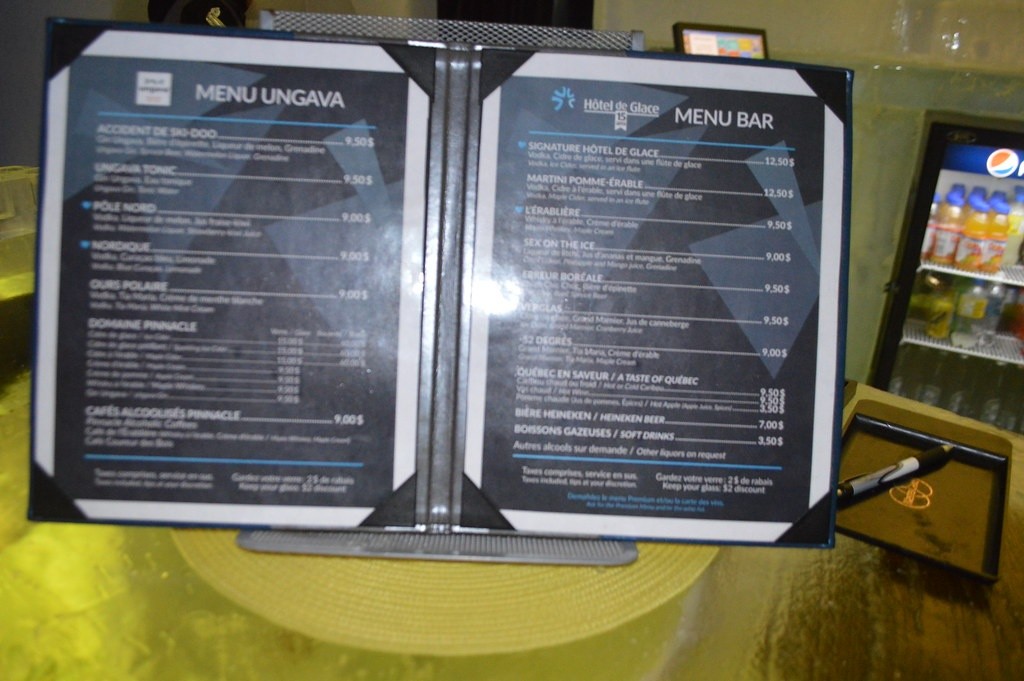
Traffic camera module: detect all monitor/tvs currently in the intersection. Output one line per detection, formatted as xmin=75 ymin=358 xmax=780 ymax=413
xmin=673 ymin=22 xmax=768 ymax=60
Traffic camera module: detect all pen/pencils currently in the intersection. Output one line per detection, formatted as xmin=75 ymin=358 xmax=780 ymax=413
xmin=837 ymin=443 xmax=955 ymax=501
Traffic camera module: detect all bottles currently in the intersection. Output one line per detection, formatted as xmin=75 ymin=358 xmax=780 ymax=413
xmin=879 ymin=183 xmax=1024 ymax=438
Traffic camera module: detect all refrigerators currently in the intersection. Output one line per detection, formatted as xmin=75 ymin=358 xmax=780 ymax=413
xmin=862 ymin=108 xmax=1023 ymax=443
